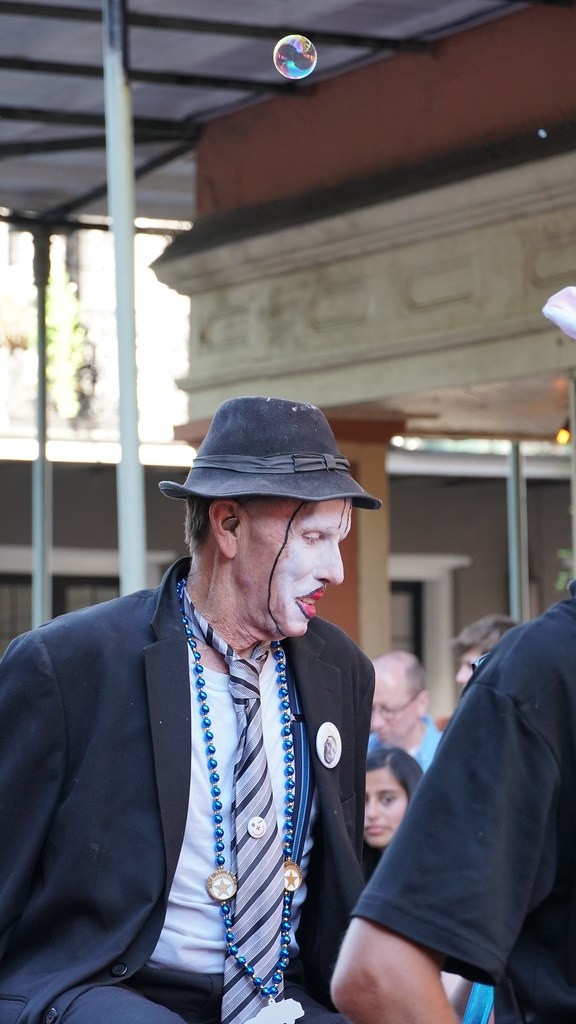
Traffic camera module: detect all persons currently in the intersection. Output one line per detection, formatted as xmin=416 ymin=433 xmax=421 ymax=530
xmin=1 ymin=397 xmax=376 ymax=1023
xmin=325 ymin=573 xmax=571 ymax=1024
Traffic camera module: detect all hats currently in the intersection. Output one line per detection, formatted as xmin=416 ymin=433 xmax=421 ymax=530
xmin=159 ymin=398 xmax=382 ymax=511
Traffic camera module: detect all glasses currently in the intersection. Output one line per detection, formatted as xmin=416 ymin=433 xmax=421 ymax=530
xmin=371 ymin=690 xmax=420 ymax=719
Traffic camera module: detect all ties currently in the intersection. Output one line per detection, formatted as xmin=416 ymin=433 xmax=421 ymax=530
xmin=176 ymin=581 xmax=283 ymax=1023
